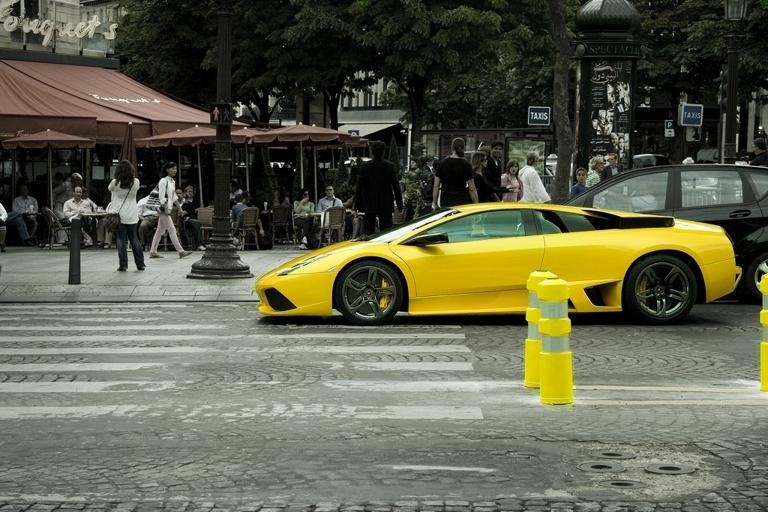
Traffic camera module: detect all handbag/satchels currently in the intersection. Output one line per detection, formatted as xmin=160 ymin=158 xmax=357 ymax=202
xmin=101 ymin=213 xmax=121 ymax=232
xmin=145 ymin=179 xmax=168 ymax=212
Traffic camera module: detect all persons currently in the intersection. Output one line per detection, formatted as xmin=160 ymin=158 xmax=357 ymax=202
xmin=755 ymin=125 xmax=768 ymax=143
xmin=409 ymin=136 xmax=623 ymax=217
xmin=150 ymin=161 xmax=192 ymax=258
xmin=750 ymin=138 xmax=768 ymax=168
xmin=603 ymin=179 xmax=659 ymax=210
xmin=481 ymin=213 xmax=517 ymax=236
xmin=682 ymin=153 xmax=695 ymax=164
xmin=592 ymin=83 xmax=629 ymax=157
xmin=352 ymin=142 xmax=404 ymax=233
xmin=685 ymin=127 xmax=701 ymax=141
xmin=105 ymin=161 xmax=147 ymax=273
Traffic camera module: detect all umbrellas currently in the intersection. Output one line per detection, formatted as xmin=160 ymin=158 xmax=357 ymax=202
xmin=118 ymin=121 xmax=140 ymax=179
xmin=0 ymin=126 xmax=96 ymax=209
xmin=135 ymin=128 xmax=184 ymax=186
xmin=231 ymin=120 xmax=370 ymax=210
xmin=150 ymin=124 xmax=246 ymax=240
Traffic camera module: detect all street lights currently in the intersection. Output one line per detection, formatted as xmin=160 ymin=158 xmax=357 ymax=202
xmin=716 ymin=0 xmax=752 ymax=166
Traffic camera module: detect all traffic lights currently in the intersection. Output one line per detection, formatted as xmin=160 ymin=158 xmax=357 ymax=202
xmin=210 ymin=102 xmax=234 ymax=124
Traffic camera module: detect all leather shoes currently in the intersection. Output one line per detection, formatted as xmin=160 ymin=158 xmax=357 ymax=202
xmin=103 ymin=242 xmax=112 ymax=248
xmin=198 ymin=245 xmax=206 ymax=251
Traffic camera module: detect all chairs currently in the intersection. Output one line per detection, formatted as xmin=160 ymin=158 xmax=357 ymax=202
xmin=0 ymin=188 xmax=407 ymax=251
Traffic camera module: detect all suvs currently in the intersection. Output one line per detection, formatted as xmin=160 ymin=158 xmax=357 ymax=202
xmin=559 ymin=162 xmax=768 ymax=304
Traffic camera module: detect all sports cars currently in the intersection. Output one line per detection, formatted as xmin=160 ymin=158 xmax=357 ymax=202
xmin=251 ymin=200 xmax=748 ymax=326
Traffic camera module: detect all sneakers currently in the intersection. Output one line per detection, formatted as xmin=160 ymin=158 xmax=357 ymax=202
xmin=138 ymin=265 xmax=145 ymax=270
xmin=23 ymin=236 xmax=39 ymax=246
xmin=299 ymin=236 xmax=308 ymax=250
xmin=117 ymin=267 xmax=126 ymax=272
xmin=179 ymin=251 xmax=192 ymax=259
xmin=149 ymin=253 xmax=164 ymax=258
